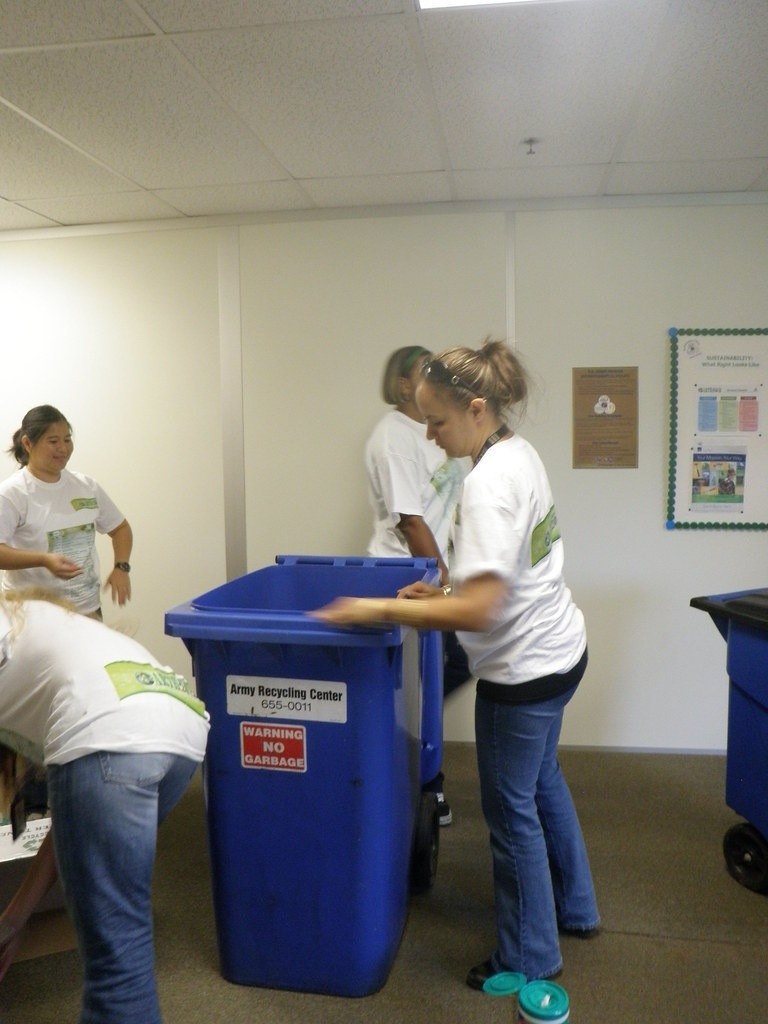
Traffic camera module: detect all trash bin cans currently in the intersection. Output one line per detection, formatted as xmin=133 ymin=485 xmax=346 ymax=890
xmin=689 ymin=585 xmax=768 ymax=896
xmin=162 ymin=552 xmax=444 ymax=1001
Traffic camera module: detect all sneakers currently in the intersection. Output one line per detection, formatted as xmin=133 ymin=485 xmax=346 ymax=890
xmin=435 ymin=785 xmax=452 ymax=825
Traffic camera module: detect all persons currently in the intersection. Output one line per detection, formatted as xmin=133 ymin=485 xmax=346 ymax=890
xmin=308 ymin=341 xmax=601 ymax=992
xmin=0 ymin=589 xmax=210 ymax=1024
xmin=719 ymin=470 xmax=735 ymax=495
xmin=364 ymin=346 xmax=471 ymax=826
xmin=0 ymin=405 xmax=133 ymax=618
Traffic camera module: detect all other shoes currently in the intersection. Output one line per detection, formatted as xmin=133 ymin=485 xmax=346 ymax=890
xmin=467 ymin=950 xmax=565 ymax=992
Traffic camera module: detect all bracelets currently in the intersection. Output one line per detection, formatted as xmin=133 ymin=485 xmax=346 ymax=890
xmin=441 ymin=584 xmax=451 ymax=595
xmin=114 ymin=562 xmax=130 ymax=571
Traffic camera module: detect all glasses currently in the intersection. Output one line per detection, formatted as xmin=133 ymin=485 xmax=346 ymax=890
xmin=418 ymin=357 xmax=483 ymax=398
